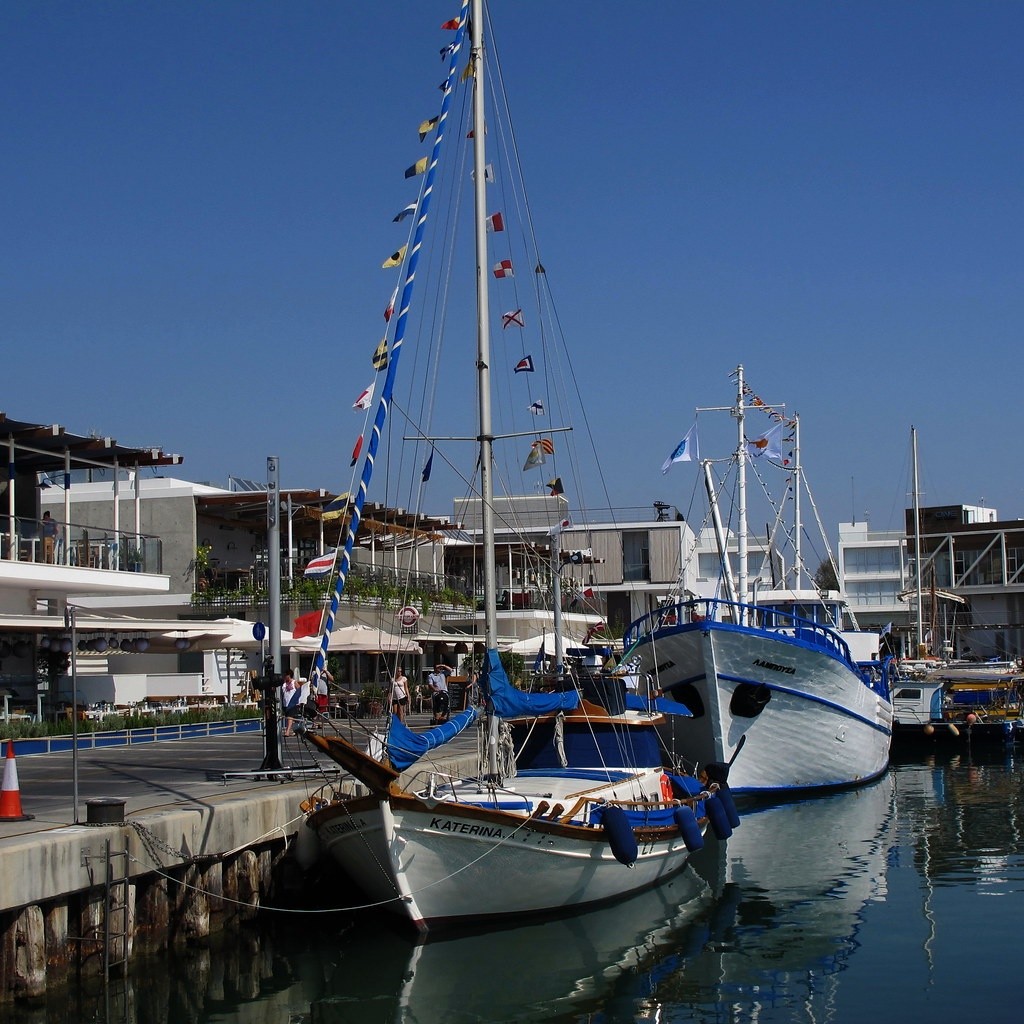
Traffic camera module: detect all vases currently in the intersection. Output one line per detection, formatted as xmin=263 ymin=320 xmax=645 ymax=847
xmin=198 ymin=577 xmax=209 ymax=592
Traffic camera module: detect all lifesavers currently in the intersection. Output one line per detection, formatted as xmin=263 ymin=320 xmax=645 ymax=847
xmin=660 ymin=774 xmax=674 ymax=808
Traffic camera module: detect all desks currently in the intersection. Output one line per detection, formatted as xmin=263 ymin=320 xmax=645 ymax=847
xmin=86 ymin=711 xmax=120 ymax=722
xmin=141 ymin=708 xmax=156 ymax=716
xmin=70 ymin=543 xmax=110 ymax=569
xmin=188 ymin=704 xmax=224 ymax=710
xmin=0 ymin=714 xmax=32 ymax=722
xmin=0 ymin=533 xmax=18 ymax=562
xmin=217 ymin=568 xmax=251 ymax=591
xmin=19 ymin=538 xmax=40 ymax=563
xmin=158 ymin=706 xmax=190 ymax=714
xmin=231 ymin=703 xmax=259 ymax=711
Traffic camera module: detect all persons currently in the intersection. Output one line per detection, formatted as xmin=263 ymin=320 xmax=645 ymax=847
xmin=312 ymin=661 xmax=333 ymax=727
xmin=37 ymin=511 xmax=59 ymax=561
xmin=428 ymin=662 xmax=454 ymax=721
xmin=388 ymin=667 xmax=409 ymax=723
xmin=465 ymin=667 xmax=478 ymax=709
xmin=283 ymin=668 xmax=300 ymax=736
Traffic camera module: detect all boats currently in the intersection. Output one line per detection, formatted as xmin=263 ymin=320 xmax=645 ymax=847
xmin=710 ymin=768 xmax=900 ymax=948
xmin=620 ymin=364 xmax=896 ymax=806
xmin=890 ymin=423 xmax=1023 ymax=735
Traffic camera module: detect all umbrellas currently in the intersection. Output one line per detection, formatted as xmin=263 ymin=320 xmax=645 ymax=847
xmin=139 ymin=615 xmax=321 ymax=707
xmin=263 ymin=620 xmax=424 ymax=693
xmin=505 ymin=631 xmax=590 ymax=660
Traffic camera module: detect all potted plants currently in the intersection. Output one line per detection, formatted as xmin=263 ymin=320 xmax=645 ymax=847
xmin=118 ymin=540 xmax=144 ymax=572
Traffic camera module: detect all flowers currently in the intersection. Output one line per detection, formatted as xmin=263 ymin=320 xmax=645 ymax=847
xmin=196 ymin=543 xmax=213 ymax=566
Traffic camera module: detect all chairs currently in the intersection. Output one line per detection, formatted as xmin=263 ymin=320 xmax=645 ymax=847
xmin=329 ymin=692 xmax=342 ymax=719
xmin=93 ymin=546 xmax=110 ymax=569
xmin=345 ymin=692 xmax=360 ymax=719
xmin=66 ymin=705 xmax=257 ymax=720
xmin=45 ymin=536 xmax=54 ymax=564
xmin=366 ymin=697 xmax=383 ymax=719
xmin=10 ymin=709 xmax=25 ymax=726
xmin=414 ymin=684 xmax=433 ymax=713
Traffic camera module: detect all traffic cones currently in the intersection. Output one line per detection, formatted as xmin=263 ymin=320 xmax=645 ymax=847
xmin=0 ymin=739 xmax=36 ymax=822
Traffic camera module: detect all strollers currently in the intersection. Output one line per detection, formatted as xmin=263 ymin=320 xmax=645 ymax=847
xmin=430 ymin=688 xmax=451 ymax=726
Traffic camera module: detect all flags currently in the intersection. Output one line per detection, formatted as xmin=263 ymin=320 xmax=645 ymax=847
xmin=534 ymin=642 xmax=544 ymax=672
xmin=728 ymin=370 xmax=797 ymax=465
xmin=293 ymin=608 xmax=322 ymax=638
xmin=879 ymin=622 xmax=891 ymax=639
xmin=660 ymin=424 xmax=694 ymax=476
xmin=544 ymin=475 xmax=583 ymax=564
xmin=568 ymin=587 xmax=605 ymax=644
xmin=441 ymin=15 xmax=553 ymax=472
xmin=291 ymin=108 xmax=440 ymax=575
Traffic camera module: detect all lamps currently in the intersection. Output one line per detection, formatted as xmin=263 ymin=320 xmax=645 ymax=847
xmin=202 ymin=538 xmax=214 ymax=550
xmin=251 ymin=544 xmax=262 ymax=555
xmin=227 ymin=541 xmax=238 ymax=553
xmin=35 ymin=473 xmax=65 ymax=490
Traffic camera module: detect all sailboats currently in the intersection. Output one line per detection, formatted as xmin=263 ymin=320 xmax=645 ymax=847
xmin=295 ymin=1 xmax=739 ymax=931
xmin=356 ymin=859 xmax=720 ymax=1024
